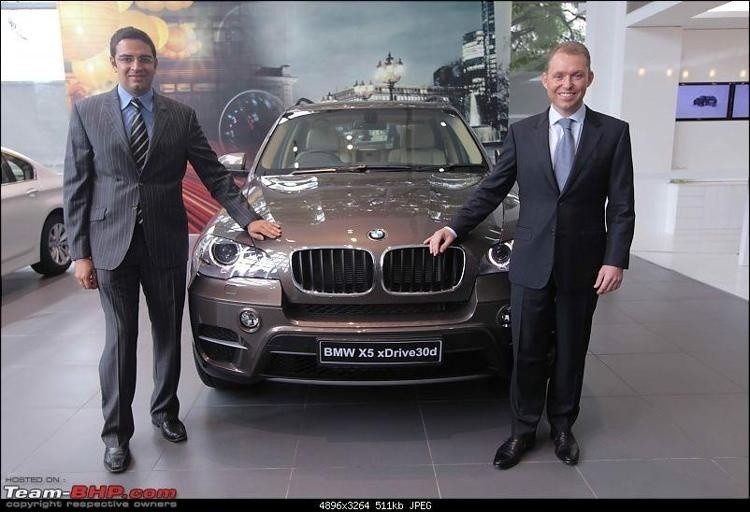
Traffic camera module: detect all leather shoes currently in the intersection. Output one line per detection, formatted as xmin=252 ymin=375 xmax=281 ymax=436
xmin=104 ymin=441 xmax=131 ymax=472
xmin=551 ymin=430 xmax=579 ymax=464
xmin=494 ymin=436 xmax=535 ymax=470
xmin=153 ymin=418 xmax=187 ymax=442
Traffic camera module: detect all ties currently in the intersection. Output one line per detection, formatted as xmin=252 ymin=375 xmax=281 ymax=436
xmin=554 ymin=119 xmax=576 ymax=194
xmin=131 ymin=99 xmax=149 ymax=225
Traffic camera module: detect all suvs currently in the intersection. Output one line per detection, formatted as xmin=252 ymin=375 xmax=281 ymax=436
xmin=188 ymin=101 xmax=522 ymax=396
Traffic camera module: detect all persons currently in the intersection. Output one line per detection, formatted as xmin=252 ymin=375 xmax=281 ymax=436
xmin=62 ymin=26 xmax=281 ymax=476
xmin=424 ymin=40 xmax=637 ymax=470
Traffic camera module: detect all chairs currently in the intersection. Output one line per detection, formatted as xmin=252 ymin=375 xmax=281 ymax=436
xmin=388 ymin=121 xmax=450 ymax=167
xmin=296 ymin=121 xmax=355 ymax=166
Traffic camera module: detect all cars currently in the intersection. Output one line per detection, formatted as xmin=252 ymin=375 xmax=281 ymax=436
xmin=1 ymin=146 xmax=73 ymax=283
xmin=692 ymin=94 xmax=718 ymax=106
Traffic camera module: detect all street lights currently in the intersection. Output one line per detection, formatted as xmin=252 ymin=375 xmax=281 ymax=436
xmin=353 ymin=79 xmax=374 ymax=99
xmin=373 ymin=51 xmax=405 ymax=99
xmin=320 ymin=93 xmax=340 ymax=101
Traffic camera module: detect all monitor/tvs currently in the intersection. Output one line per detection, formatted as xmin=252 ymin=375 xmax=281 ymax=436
xmin=676 ymin=82 xmax=732 ymax=121
xmin=729 ymin=82 xmax=750 ymax=120
